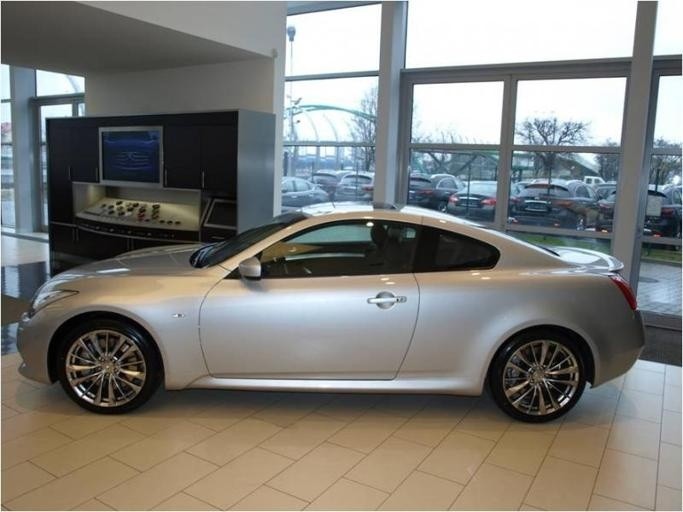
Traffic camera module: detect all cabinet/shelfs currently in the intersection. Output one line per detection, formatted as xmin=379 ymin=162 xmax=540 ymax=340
xmin=45 ymin=107 xmax=276 ymax=265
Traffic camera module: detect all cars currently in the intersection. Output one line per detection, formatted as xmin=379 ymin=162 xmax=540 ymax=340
xmin=16 ymin=200 xmax=646 ymax=423
xmin=281 ymin=171 xmax=682 ymax=252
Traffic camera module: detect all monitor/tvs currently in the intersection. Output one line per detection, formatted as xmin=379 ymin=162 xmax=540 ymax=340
xmin=98 ymin=126 xmax=162 ymax=189
xmin=203 ymin=198 xmax=236 ymax=230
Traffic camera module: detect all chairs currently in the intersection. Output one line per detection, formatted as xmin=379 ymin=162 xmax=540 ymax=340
xmin=363 ymin=224 xmax=384 ymax=265
xmin=379 ymin=230 xmax=402 ymax=273
